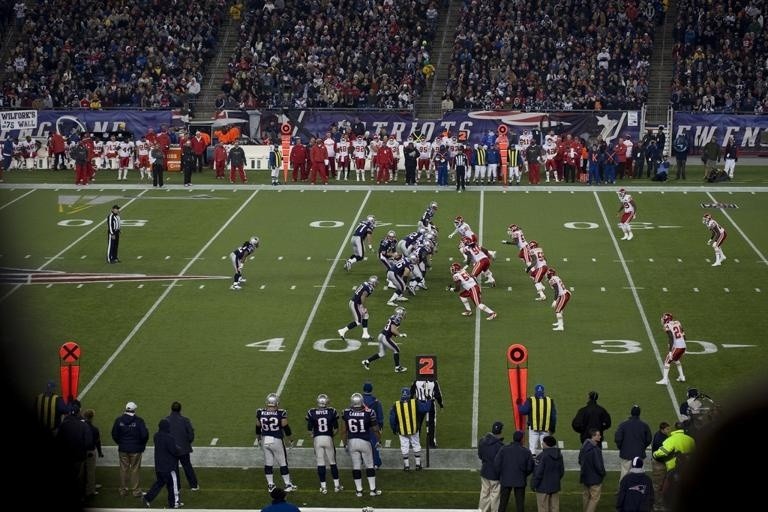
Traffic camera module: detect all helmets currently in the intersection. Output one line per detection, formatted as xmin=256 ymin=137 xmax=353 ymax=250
xmin=369 ymin=276 xmax=377 ymax=287
xmin=249 ymin=236 xmax=259 ymax=248
xmin=615 ymin=190 xmax=627 ymax=199
xmin=386 ymin=202 xmax=438 ymax=264
xmin=265 ymin=384 xmax=409 ymax=408
xmin=394 ymin=307 xmax=406 ymax=320
xmin=509 ymin=225 xmax=555 ymax=280
xmin=367 ymin=215 xmax=375 ymax=223
xmin=450 ymin=214 xmax=476 ymax=275
xmin=661 ymin=312 xmax=673 ymax=325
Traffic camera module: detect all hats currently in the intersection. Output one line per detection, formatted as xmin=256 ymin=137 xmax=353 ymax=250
xmin=493 ymin=385 xmax=698 ymax=468
xmin=113 ymin=205 xmax=120 ymax=209
xmin=47 ymin=381 xmax=56 ymax=388
xmin=125 ymin=402 xmax=136 ymax=411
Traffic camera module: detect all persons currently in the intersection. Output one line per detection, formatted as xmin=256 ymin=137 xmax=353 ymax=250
xmin=390 ymin=377 xmax=444 ymax=471
xmin=1 ymin=1 xmax=767 ymax=117
xmin=362 ymin=307 xmax=407 ymax=372
xmin=260 ymin=488 xmax=300 ymax=512
xmin=32 ymin=381 xmax=104 ymax=496
xmin=2 ymin=120 xmax=738 ymax=191
xmin=0 ymin=256 xmax=91 ymax=512
xmin=337 ymin=276 xmax=380 ymax=342
xmin=256 ymin=393 xmax=297 ymax=493
xmin=547 ymin=268 xmax=572 ymax=332
xmin=655 ymin=312 xmax=687 ymax=384
xmin=616 ymin=189 xmax=637 ymax=240
xmin=228 ymin=237 xmax=262 ymax=290
xmin=525 ymin=240 xmax=548 ymax=301
xmin=144 ymin=401 xmax=200 ymax=509
xmin=499 ymin=224 xmax=529 ymax=263
xmin=344 ymin=214 xmax=376 ymax=272
xmin=105 ymin=205 xmax=123 ymax=265
xmin=306 ymin=383 xmax=384 ymax=497
xmin=478 ymin=385 xmax=768 ymax=512
xmin=378 ymin=199 xmax=497 ymax=321
xmin=109 ymin=402 xmax=149 ymax=497
xmin=702 ymin=213 xmax=727 ymax=267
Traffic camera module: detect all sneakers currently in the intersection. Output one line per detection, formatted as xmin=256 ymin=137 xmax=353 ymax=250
xmin=344 ymin=260 xmax=351 ymax=272
xmin=620 ymin=232 xmax=628 ymax=240
xmin=553 ymin=327 xmax=564 ymax=331
xmin=628 ymin=233 xmax=633 ymax=241
xmin=656 ymin=381 xmax=669 ymax=386
xmin=238 ymin=277 xmax=246 ymax=282
xmin=387 ymin=282 xmax=427 ymax=307
xmin=462 ymin=310 xmax=472 ymax=316
xmin=677 ymin=377 xmax=685 ymax=381
xmin=337 ymin=327 xmax=407 ymax=374
xmin=484 ymin=277 xmax=496 ymax=283
xmin=712 ymin=263 xmax=721 ymax=267
xmin=720 ymin=256 xmax=726 ymax=262
xmin=486 ymin=313 xmax=497 ymax=320
xmin=231 ymin=283 xmax=243 ymax=290
xmin=552 ymin=322 xmax=559 ymax=326
xmin=268 ymin=464 xmax=422 ymax=497
